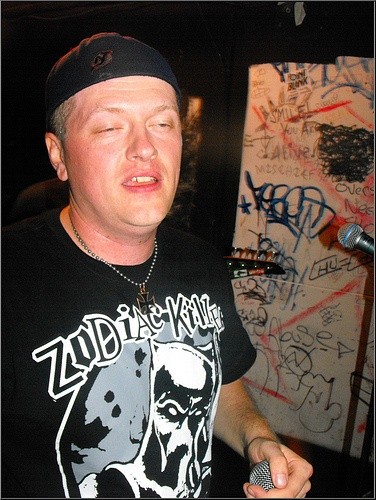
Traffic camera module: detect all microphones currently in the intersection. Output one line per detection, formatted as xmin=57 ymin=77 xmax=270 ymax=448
xmin=337 ymin=223 xmax=374 ymax=258
xmin=249 ymin=460 xmax=277 ymax=492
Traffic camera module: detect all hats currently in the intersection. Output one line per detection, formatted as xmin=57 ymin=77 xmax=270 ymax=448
xmin=44 ymin=31 xmax=183 ymax=128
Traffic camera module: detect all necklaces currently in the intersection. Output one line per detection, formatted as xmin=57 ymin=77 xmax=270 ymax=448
xmin=67 ymin=210 xmax=161 ymax=320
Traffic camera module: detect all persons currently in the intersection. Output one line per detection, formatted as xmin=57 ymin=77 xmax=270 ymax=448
xmin=0 ymin=34 xmax=314 ymax=498
